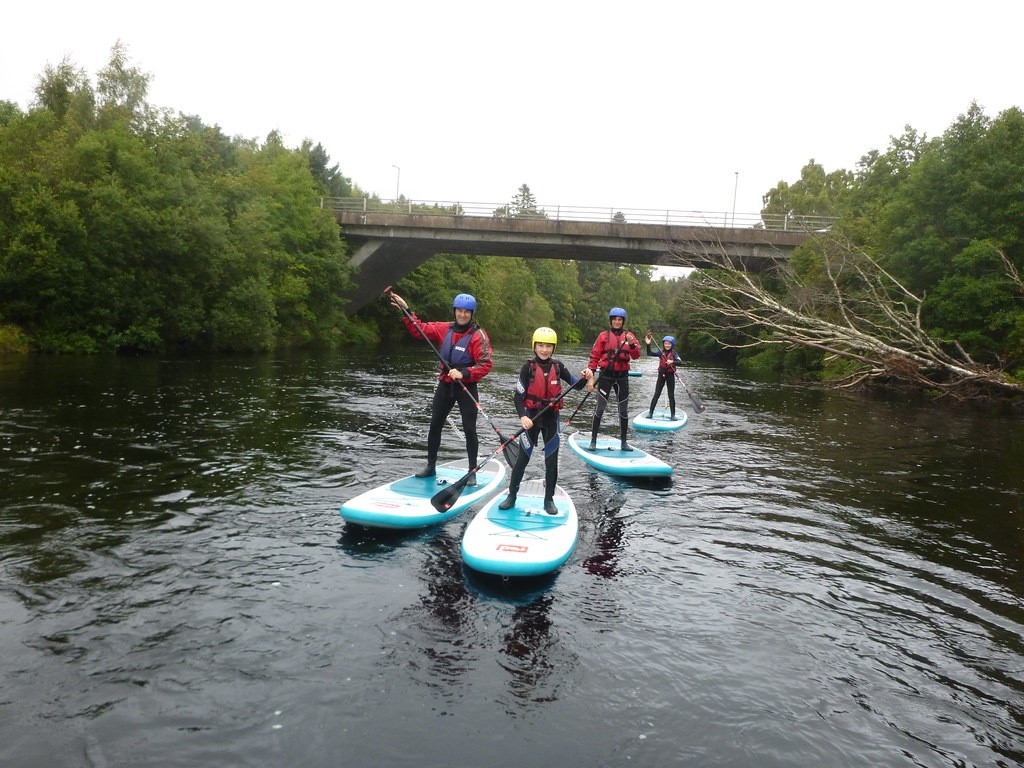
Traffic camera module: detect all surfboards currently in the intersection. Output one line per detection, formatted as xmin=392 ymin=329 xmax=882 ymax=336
xmin=568 ymin=430 xmax=675 ymax=481
xmin=340 ymin=456 xmax=506 ymax=531
xmin=632 ymin=405 xmax=688 ymax=431
xmin=460 ymin=477 xmax=581 ymax=576
xmin=628 ymin=371 xmax=643 ymax=376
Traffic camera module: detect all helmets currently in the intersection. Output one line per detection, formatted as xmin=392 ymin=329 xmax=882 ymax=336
xmin=531 ymin=327 xmax=557 ymax=353
xmin=453 ymin=294 xmax=476 ymax=316
xmin=662 ymin=336 xmax=676 ymax=348
xmin=609 ymin=308 xmax=627 ymax=323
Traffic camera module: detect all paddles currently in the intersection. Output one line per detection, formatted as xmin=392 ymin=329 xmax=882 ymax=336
xmin=648 ymin=333 xmax=707 ymax=415
xmin=382 ymin=284 xmax=518 ymax=468
xmin=430 ymin=377 xmax=583 ymax=514
xmin=566 ymin=339 xmax=626 ymax=425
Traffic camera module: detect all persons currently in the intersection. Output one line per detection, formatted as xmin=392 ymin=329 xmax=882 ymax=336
xmin=498 ymin=327 xmax=594 ymax=514
xmin=391 ymin=292 xmax=493 ymax=486
xmin=588 ymin=308 xmax=641 ymax=451
xmin=645 ymin=335 xmax=683 ymax=421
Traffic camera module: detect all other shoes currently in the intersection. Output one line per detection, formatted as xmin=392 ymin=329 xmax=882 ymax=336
xmin=544 ymin=498 xmax=558 ymax=514
xmin=466 ymin=473 xmax=476 ymax=486
xmin=416 ymin=465 xmax=436 ymax=477
xmin=670 ymin=415 xmax=678 ymax=421
xmin=645 ymin=412 xmax=653 ymax=418
xmin=621 ymin=442 xmax=633 ymax=451
xmin=499 ymin=497 xmax=515 ymax=509
xmin=589 ymin=440 xmax=596 ymax=450
